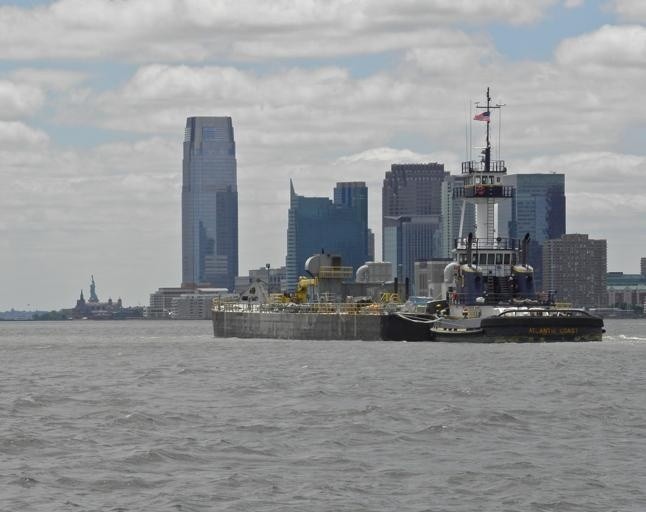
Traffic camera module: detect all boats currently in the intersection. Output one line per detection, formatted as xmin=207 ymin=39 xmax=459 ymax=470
xmin=428 ymin=82 xmax=606 ymax=344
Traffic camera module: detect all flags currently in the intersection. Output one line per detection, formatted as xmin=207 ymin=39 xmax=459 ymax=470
xmin=473 ymin=111 xmax=490 ymax=123
